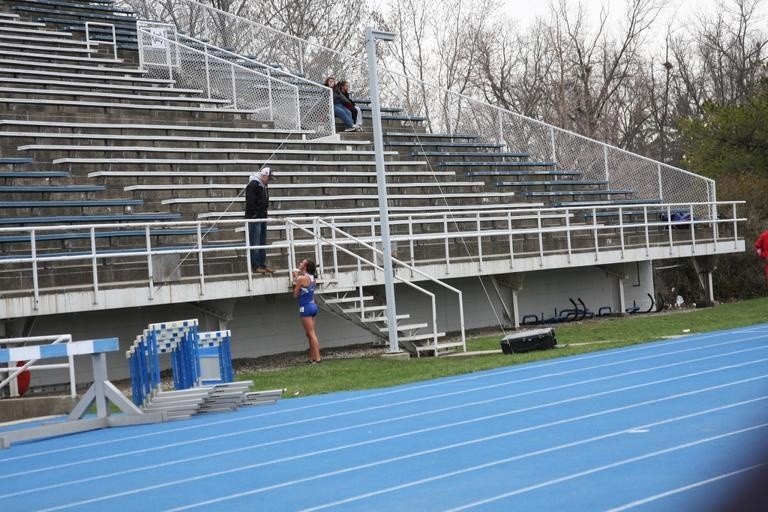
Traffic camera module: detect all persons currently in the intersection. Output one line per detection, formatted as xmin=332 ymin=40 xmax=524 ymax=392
xmin=243 ymin=165 xmax=277 ymax=273
xmin=320 ymin=76 xmax=360 ymax=133
xmin=336 ymin=82 xmax=365 ymax=132
xmin=753 ymin=229 xmax=767 ymax=286
xmin=292 ymin=258 xmax=321 ymax=363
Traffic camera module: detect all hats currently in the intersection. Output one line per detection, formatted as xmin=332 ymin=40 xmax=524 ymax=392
xmin=261 ymin=167 xmax=278 ymax=180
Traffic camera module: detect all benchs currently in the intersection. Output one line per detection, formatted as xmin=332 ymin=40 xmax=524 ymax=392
xmin=0 ymin=0 xmax=715 ymax=296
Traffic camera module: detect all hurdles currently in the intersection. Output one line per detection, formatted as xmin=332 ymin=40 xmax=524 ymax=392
xmin=0 ymin=338 xmax=167 ymax=449
xmin=125 ymin=318 xmax=285 ymax=420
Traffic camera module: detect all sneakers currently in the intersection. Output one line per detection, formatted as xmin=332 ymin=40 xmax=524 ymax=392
xmin=256 ymin=266 xmax=275 ymax=274
xmin=309 ymin=360 xmax=324 ymax=365
xmin=345 ymin=125 xmax=364 ymax=132
